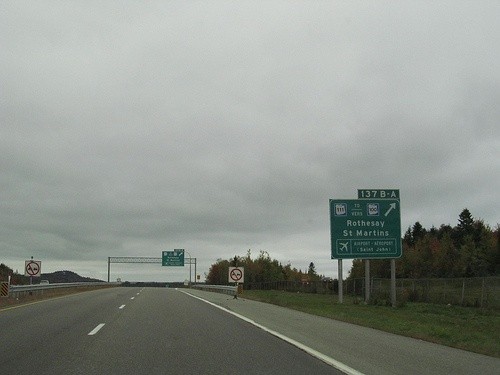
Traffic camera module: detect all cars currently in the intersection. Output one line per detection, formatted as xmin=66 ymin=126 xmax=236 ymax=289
xmin=40 ymin=280 xmax=49 ymax=284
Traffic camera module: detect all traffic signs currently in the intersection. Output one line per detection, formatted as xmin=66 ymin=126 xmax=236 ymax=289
xmin=328 ymin=198 xmax=402 ymax=260
xmin=162 ymin=251 xmax=185 ymax=266
